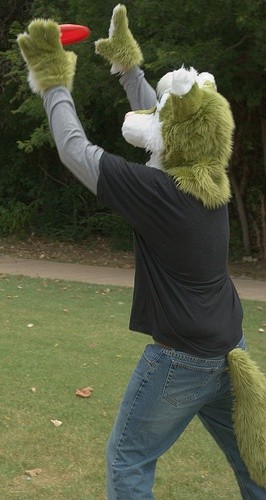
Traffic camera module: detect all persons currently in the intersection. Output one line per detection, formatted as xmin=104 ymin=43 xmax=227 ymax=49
xmin=15 ymin=3 xmax=266 ymax=500
